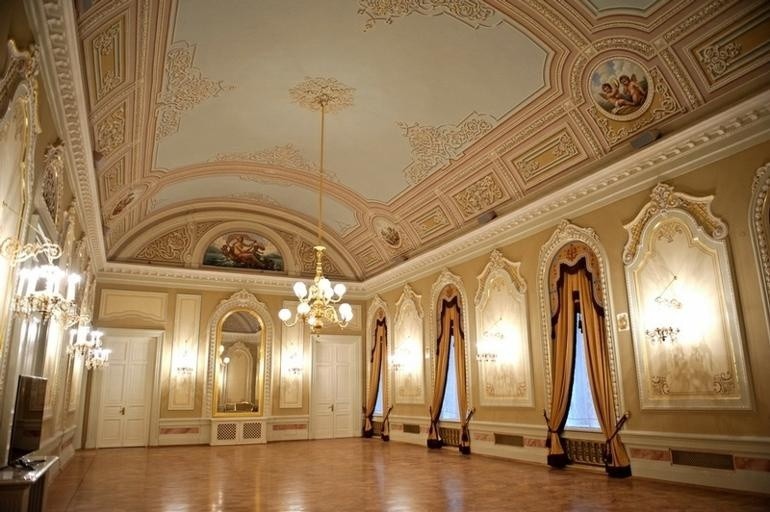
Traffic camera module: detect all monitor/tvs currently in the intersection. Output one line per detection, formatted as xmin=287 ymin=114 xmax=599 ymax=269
xmin=9 ymin=373 xmax=48 ymax=472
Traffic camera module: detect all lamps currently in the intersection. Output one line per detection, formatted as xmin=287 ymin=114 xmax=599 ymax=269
xmin=0 ymin=200 xmax=82 ymax=329
xmin=474 ymin=317 xmax=503 ymax=360
xmin=276 ymin=75 xmax=353 ymax=331
xmin=640 ymin=275 xmax=683 ymax=344
xmin=219 ymin=345 xmax=230 ymax=369
xmin=67 ymin=314 xmax=112 ymax=371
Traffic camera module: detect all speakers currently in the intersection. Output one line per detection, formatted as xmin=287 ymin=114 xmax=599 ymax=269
xmin=631 ymin=131 xmax=658 ymax=149
xmin=478 ymin=211 xmax=496 ymax=226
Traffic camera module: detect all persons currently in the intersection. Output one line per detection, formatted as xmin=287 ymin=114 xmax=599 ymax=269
xmin=600 ymin=81 xmax=635 ymax=108
xmin=620 ymin=74 xmax=646 ymax=106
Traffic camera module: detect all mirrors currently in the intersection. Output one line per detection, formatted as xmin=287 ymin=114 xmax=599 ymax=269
xmin=199 ymin=289 xmax=275 ymax=419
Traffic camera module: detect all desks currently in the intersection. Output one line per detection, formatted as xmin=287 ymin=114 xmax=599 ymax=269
xmin=0 ymin=454 xmax=59 ymax=512
